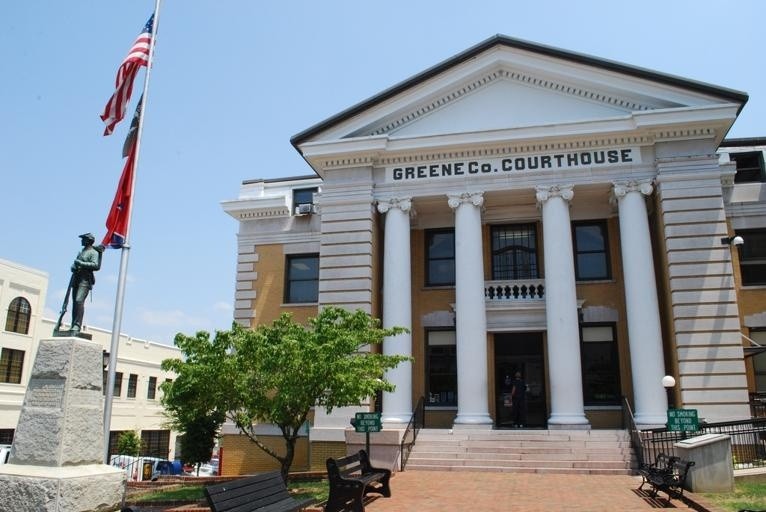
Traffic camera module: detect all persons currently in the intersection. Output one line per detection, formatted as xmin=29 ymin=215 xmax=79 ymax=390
xmin=51 ymin=232 xmax=99 ymax=331
xmin=510 ymin=371 xmax=527 ymax=428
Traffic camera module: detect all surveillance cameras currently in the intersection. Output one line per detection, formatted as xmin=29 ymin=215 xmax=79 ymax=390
xmin=734 ymin=236 xmax=744 ymax=249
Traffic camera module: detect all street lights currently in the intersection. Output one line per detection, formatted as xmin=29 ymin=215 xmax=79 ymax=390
xmin=661 ymin=371 xmax=687 ymax=441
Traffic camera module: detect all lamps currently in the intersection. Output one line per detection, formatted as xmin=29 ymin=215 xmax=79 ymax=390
xmin=720 ymin=233 xmax=744 ymax=247
xmin=663 ymin=372 xmax=676 ymax=410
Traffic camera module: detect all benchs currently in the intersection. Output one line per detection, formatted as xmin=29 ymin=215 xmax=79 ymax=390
xmin=325 ymin=448 xmax=392 ymax=512
xmin=204 ymin=471 xmax=315 ymax=511
xmin=636 ymin=452 xmax=696 ymax=509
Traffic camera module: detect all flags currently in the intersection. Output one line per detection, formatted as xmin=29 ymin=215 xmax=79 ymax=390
xmin=98 ymin=0 xmax=158 ymax=137
xmin=98 ymin=88 xmax=144 ymax=248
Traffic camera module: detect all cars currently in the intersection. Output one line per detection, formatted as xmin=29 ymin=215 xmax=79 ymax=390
xmin=106 ymin=451 xmax=221 ymax=482
